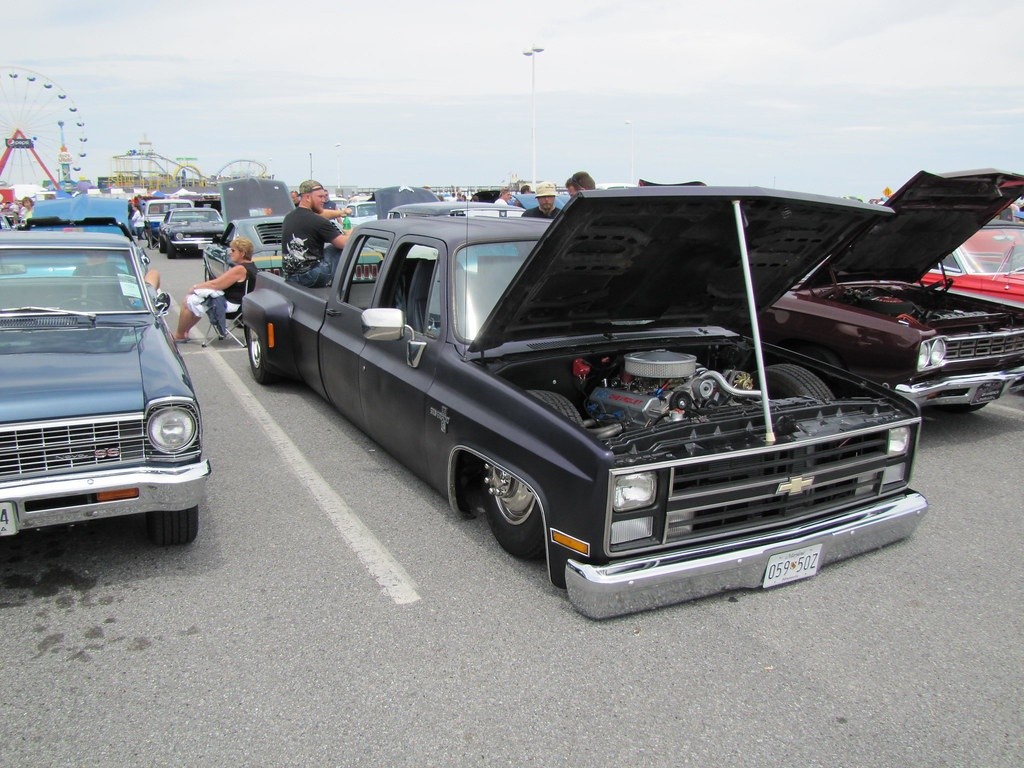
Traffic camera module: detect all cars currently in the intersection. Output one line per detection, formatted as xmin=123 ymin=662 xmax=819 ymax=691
xmin=373 ymin=187 xmax=529 ymax=274
xmin=913 ymin=219 xmax=1024 ymax=311
xmin=22 ymin=196 xmax=150 ymax=281
xmin=159 ymin=208 xmax=228 ymax=259
xmin=241 ymin=185 xmax=932 ymax=623
xmin=335 ymin=202 xmax=377 ymax=231
xmin=738 ymin=167 xmax=1024 ymax=420
xmin=0 ymin=230 xmax=211 ymax=546
xmin=140 ymin=200 xmax=194 ymax=243
xmin=329 ymin=198 xmax=347 ymax=209
xmin=201 ymin=177 xmax=382 ymax=301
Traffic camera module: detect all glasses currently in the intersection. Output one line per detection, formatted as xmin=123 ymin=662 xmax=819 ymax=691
xmin=571 ymin=177 xmax=584 ymax=188
xmin=325 ymin=194 xmax=328 ymax=196
xmin=231 ymin=248 xmax=238 ymax=253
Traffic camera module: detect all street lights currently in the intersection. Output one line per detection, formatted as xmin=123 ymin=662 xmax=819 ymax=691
xmin=523 ymin=42 xmax=544 ymax=191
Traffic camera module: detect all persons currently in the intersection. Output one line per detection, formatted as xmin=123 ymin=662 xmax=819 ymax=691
xmin=9 ymin=201 xmax=20 ymax=214
xmin=449 ymin=192 xmax=467 ymax=202
xmin=291 ymin=190 xmax=300 ymax=209
xmin=494 ymin=190 xmax=510 ymax=205
xmin=281 ymin=180 xmax=353 ymax=288
xmin=173 ymin=239 xmax=257 ymax=344
xmin=128 ymin=195 xmax=145 ymax=240
xmin=73 ymin=265 xmax=160 ymax=290
xmin=566 ymin=172 xmax=595 ymax=197
xmin=522 ymin=182 xmax=560 ymax=219
xmin=323 ymin=190 xmax=342 ymax=223
xmin=514 ymin=185 xmax=531 ymax=207
xmin=21 ymin=197 xmax=34 ymax=229
xmin=2 ymin=201 xmax=11 ymax=211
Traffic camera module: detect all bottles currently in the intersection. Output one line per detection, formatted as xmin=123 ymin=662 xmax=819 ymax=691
xmin=343 ymin=214 xmax=352 ymax=229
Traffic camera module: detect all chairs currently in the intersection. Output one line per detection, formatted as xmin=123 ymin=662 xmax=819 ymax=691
xmin=71 ymin=265 xmax=124 ymax=312
xmin=407 ymin=259 xmax=475 ymax=334
xmin=202 ymin=248 xmax=250 ymax=350
xmin=144 ymin=221 xmax=161 ymax=251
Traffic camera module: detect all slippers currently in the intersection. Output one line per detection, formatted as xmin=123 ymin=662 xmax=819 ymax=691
xmin=184 ymin=337 xmax=190 ymax=341
xmin=172 ymin=334 xmax=186 ymax=343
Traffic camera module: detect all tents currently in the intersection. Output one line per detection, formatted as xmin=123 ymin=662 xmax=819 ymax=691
xmin=153 ymin=188 xmax=200 ymax=199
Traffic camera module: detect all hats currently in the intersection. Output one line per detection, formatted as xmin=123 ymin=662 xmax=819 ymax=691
xmin=296 ymin=179 xmax=324 ymax=197
xmin=1013 ymin=212 xmax=1024 ymax=219
xmin=534 ymin=182 xmax=559 ymax=198
